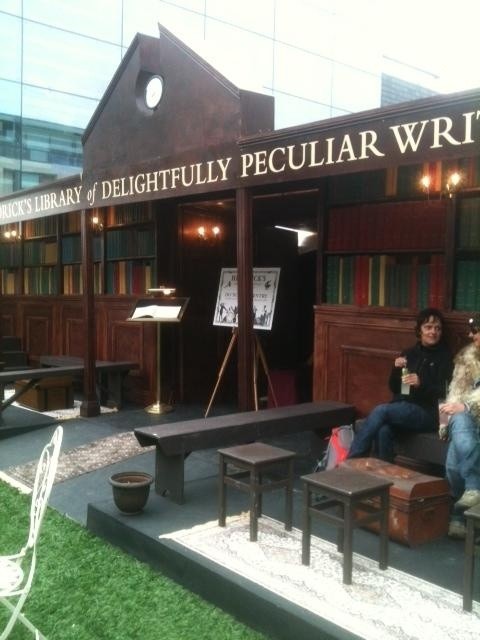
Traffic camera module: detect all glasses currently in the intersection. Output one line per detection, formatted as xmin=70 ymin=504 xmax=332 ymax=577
xmin=471 ymin=328 xmax=480 ymax=334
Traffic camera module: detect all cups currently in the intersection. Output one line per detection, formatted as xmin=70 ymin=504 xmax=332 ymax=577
xmin=438 ymin=398 xmax=450 ymax=427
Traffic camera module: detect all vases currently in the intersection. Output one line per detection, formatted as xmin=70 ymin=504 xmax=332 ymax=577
xmin=108 ymin=471 xmax=154 ymax=516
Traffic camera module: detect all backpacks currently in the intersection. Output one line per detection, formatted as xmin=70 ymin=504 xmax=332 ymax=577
xmin=311 ymin=424 xmax=356 ymax=501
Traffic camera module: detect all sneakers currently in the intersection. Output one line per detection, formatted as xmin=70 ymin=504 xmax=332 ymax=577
xmin=454 ymin=489 xmax=480 ymax=510
xmin=447 ymin=520 xmax=467 ymax=538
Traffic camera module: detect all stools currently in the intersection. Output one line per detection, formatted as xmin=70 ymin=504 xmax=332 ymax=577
xmin=217 ymin=442 xmax=297 ymax=541
xmin=300 ymin=467 xmax=395 ymax=585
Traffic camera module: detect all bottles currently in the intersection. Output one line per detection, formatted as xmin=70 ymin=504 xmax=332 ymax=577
xmin=398 ymin=368 xmax=413 ymax=401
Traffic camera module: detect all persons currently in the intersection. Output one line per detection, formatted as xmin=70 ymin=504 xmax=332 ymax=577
xmin=347 ymin=305 xmax=453 ymax=462
xmin=440 ymin=313 xmax=480 ymax=539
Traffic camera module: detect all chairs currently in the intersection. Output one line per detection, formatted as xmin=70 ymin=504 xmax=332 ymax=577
xmin=0 ymin=425 xmax=63 ymax=640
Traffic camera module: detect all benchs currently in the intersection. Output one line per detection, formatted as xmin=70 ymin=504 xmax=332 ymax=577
xmin=355 ymin=417 xmax=451 ymax=465
xmin=134 ymin=399 xmax=356 ymax=505
xmin=0 ymin=356 xmax=141 ymax=413
